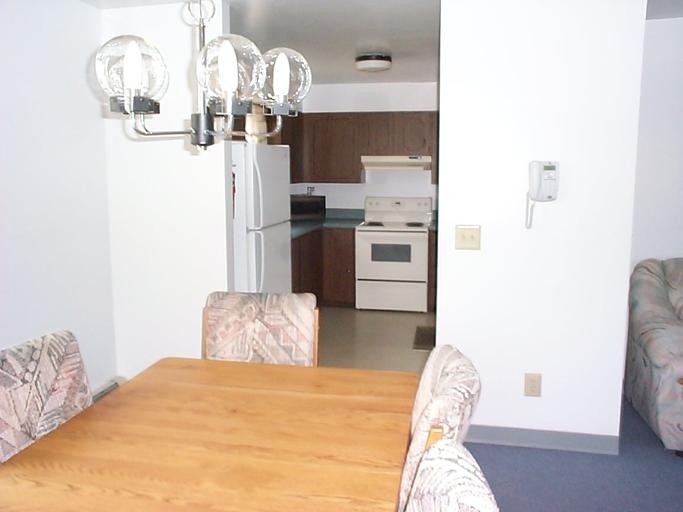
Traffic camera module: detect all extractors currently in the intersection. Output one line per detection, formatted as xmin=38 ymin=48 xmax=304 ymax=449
xmin=360 ymin=155 xmax=432 ymax=172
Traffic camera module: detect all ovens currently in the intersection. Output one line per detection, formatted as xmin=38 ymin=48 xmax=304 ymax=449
xmin=353 ymin=231 xmax=428 ymax=314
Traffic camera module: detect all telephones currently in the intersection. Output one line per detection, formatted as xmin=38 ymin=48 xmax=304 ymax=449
xmin=529 ymin=161 xmax=559 ymax=202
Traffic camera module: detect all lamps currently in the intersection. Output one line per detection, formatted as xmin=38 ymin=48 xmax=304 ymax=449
xmin=89 ymin=0 xmax=318 ymax=153
xmin=351 ymin=53 xmax=392 ymax=73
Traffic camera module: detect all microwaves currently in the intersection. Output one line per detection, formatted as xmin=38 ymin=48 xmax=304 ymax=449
xmin=289 ymin=196 xmax=326 ymax=220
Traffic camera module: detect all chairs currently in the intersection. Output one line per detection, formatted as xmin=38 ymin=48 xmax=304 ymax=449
xmin=400 ymin=423 xmax=500 ymax=511
xmin=397 ymin=342 xmax=483 ymax=510
xmin=0 ymin=327 xmax=95 ymax=467
xmin=202 ymin=290 xmax=318 ymax=370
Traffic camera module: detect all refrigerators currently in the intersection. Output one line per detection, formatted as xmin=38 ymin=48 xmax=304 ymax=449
xmin=229 ymin=138 xmax=294 ymax=294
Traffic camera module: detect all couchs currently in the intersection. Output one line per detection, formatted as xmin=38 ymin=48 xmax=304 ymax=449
xmin=625 ymin=253 xmax=683 ymax=460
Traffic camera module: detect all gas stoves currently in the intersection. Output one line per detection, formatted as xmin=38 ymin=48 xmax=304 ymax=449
xmin=354 ymin=218 xmax=426 ymax=231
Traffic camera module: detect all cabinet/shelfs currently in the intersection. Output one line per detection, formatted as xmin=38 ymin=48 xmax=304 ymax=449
xmin=288 ymin=221 xmax=354 ymax=309
xmin=212 ymin=103 xmax=437 ymax=186
